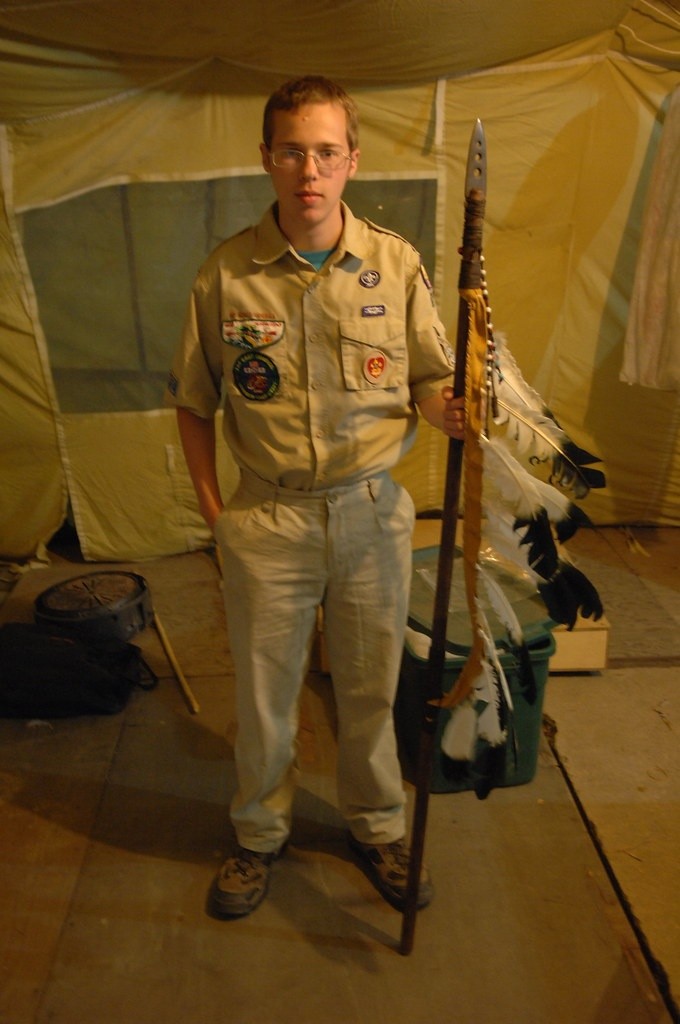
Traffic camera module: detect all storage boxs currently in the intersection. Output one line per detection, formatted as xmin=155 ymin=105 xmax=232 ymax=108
xmin=395 ymin=544 xmax=553 ymax=798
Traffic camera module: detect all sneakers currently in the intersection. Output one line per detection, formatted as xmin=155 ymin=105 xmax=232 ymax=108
xmin=211 ymin=840 xmax=288 ymax=920
xmin=348 ymin=833 xmax=433 ymax=912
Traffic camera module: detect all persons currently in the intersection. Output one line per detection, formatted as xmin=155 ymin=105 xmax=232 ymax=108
xmin=166 ymin=75 xmax=467 ymax=921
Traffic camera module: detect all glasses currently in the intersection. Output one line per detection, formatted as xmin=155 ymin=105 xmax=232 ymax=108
xmin=267 ymin=149 xmax=352 ymax=171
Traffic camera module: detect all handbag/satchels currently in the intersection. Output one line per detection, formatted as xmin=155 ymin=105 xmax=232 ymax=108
xmin=0 ymin=621 xmax=159 ymax=721
